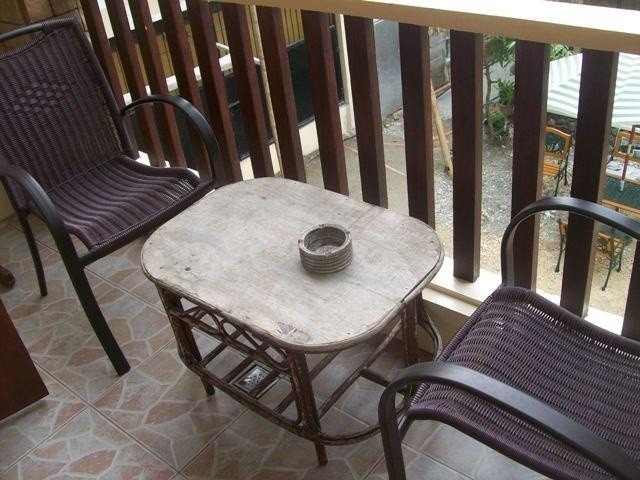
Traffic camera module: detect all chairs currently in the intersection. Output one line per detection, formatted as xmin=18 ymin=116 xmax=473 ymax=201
xmin=540 ymin=122 xmax=640 ymax=291
xmin=1 ymin=21 xmax=228 ymax=377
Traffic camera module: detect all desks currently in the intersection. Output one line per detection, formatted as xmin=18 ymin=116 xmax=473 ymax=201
xmin=142 ymin=173 xmax=443 ymax=467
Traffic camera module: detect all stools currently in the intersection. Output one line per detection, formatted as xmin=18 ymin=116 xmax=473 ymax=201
xmin=366 ymin=195 xmax=640 ymax=476
